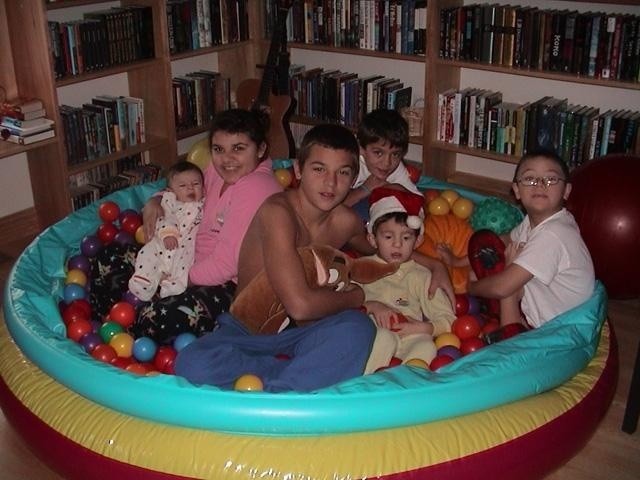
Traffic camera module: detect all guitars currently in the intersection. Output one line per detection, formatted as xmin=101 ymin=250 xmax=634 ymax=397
xmin=236 ymin=0 xmax=297 ymax=160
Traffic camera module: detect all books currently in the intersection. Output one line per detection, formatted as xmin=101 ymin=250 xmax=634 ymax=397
xmin=0 ymin=95 xmax=56 ymax=146
xmin=267 ymin=1 xmax=640 ymax=169
xmin=46 ymin=1 xmax=250 ymax=211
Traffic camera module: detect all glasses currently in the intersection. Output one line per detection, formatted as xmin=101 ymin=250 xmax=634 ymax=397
xmin=516 ymin=175 xmax=567 ymax=186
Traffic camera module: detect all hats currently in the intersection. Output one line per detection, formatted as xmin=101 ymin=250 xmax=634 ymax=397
xmin=367 ymin=182 xmax=425 ymax=238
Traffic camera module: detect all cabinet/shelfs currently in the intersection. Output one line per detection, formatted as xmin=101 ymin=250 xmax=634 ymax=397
xmin=256 ymin=0 xmax=640 ymax=203
xmin=0 ymin=0 xmax=256 ymax=224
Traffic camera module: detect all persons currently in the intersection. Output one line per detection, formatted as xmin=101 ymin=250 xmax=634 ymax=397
xmin=437 ymin=147 xmax=597 ymax=331
xmin=83 ymin=105 xmax=458 ymax=390
